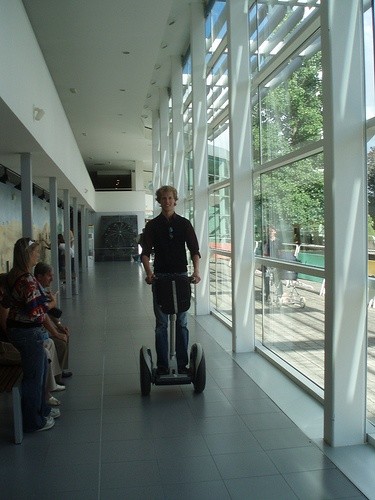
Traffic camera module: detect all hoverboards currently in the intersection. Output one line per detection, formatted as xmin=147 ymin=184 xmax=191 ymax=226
xmin=140 ymin=277 xmax=207 ymax=396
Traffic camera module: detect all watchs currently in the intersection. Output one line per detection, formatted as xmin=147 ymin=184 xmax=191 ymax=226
xmin=58 ymin=321 xmax=61 ymax=324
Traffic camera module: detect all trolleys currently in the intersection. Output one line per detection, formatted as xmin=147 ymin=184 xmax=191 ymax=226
xmin=275 ymin=255 xmax=305 ymax=310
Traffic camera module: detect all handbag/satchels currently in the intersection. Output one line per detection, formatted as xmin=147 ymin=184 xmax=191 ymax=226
xmin=49 ymin=307 xmax=63 ymax=318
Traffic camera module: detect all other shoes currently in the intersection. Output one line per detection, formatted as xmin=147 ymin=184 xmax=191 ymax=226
xmin=56 ymin=375 xmax=64 ymax=384
xmin=37 ymin=416 xmax=55 ymax=431
xmin=50 ymin=384 xmax=66 ymax=391
xmin=178 ymin=367 xmax=191 ymax=374
xmin=48 ymin=407 xmax=60 ymax=418
xmin=48 ymin=396 xmax=60 ymax=405
xmin=152 ymin=367 xmax=170 ymax=376
xmin=62 ymin=369 xmax=73 ymax=377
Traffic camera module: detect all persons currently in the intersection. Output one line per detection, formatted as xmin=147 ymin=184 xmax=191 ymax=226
xmin=0 ymin=237 xmax=73 ymax=433
xmin=137 ymin=185 xmax=202 ymax=376
xmin=261 ymin=226 xmax=284 ymax=302
xmin=42 ymin=230 xmax=75 ymax=272
xmin=131 ymin=242 xmax=138 ymax=262
